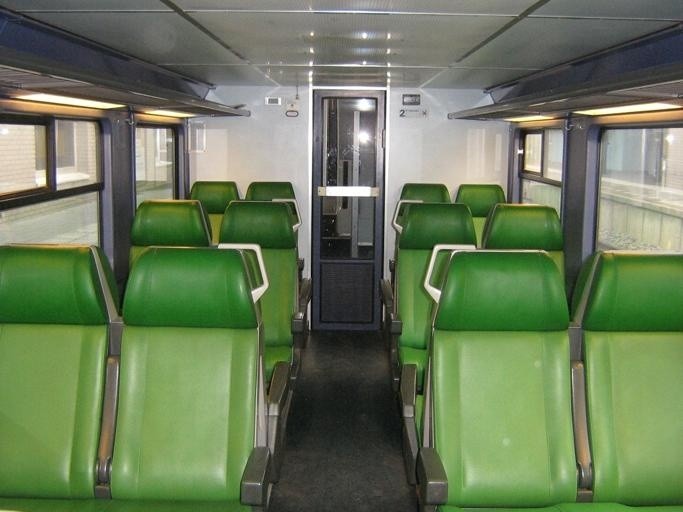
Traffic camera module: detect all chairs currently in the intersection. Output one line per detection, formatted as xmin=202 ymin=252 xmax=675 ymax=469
xmin=379 ymin=184 xmax=683 ymax=512
xmin=0 ymin=180 xmax=313 ymax=512
